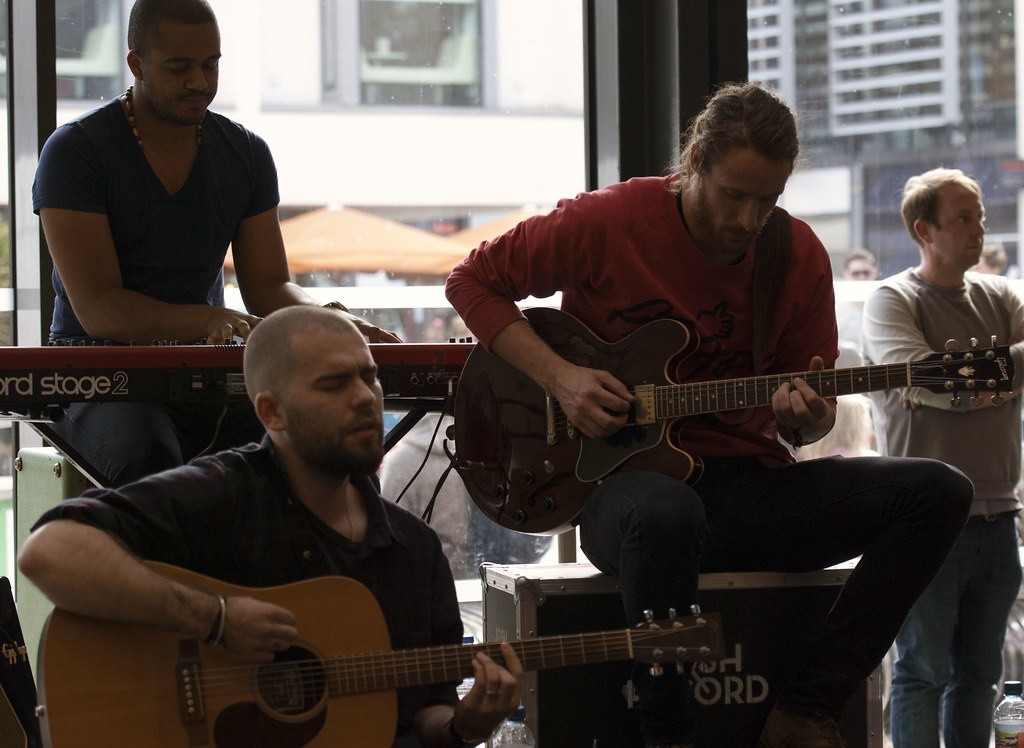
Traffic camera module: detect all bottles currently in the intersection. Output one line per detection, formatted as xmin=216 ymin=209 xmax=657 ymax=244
xmin=456 ymin=635 xmax=475 ymax=700
xmin=493 ymin=705 xmax=536 ymax=747
xmin=993 ymin=680 xmax=1024 ymax=748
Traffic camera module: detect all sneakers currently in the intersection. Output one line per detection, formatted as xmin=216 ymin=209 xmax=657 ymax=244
xmin=759 ymin=706 xmax=847 ymax=748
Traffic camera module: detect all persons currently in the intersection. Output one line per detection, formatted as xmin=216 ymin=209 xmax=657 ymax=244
xmin=17 ymin=305 xmax=524 ymax=748
xmin=798 ymin=167 xmax=1024 ymax=748
xmin=33 ymin=0 xmax=402 ymax=491
xmin=444 ymin=84 xmax=974 ymax=748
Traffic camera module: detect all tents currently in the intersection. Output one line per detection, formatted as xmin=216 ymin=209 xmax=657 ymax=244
xmin=222 ymin=206 xmax=548 ymax=277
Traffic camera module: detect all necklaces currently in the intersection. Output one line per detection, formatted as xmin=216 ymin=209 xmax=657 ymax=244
xmin=343 ymin=483 xmax=356 ymax=543
xmin=125 ymin=86 xmax=206 ymax=192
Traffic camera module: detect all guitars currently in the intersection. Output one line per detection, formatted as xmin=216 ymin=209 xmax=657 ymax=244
xmin=453 ymin=304 xmax=1020 ymax=539
xmin=33 ymin=557 xmax=732 ymax=747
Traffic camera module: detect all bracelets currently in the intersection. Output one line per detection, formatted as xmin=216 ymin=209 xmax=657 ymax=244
xmin=207 ymin=593 xmax=227 ymax=647
xmin=207 ymin=609 xmax=220 ymax=644
xmin=448 ymin=718 xmax=490 ymax=745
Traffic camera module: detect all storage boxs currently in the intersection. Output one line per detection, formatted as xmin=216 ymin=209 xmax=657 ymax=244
xmin=478 ymin=561 xmax=886 ymax=748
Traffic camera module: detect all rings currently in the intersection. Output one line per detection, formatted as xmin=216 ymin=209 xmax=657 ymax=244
xmin=487 ymin=689 xmax=502 ymax=695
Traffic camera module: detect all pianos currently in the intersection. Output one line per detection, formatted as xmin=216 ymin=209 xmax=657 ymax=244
xmin=0 ymin=334 xmax=480 ymax=492
